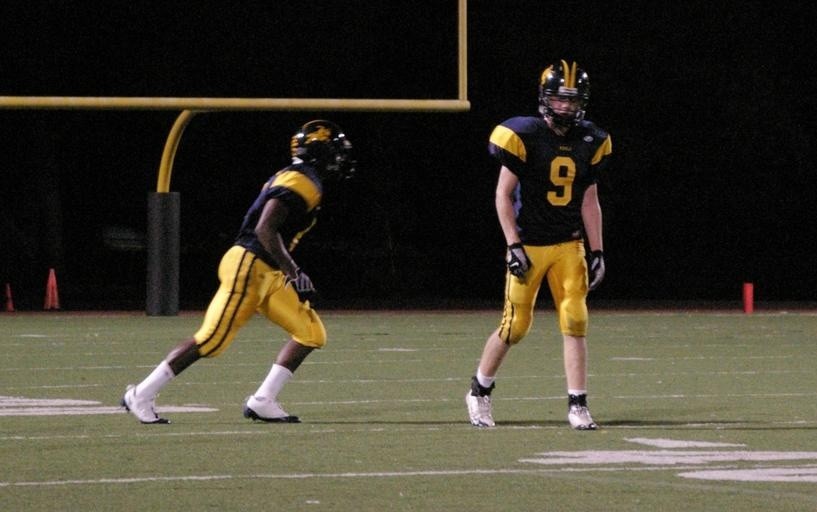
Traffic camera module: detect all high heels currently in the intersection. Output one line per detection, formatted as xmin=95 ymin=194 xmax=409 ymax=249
xmin=0 ymin=283 xmax=14 ymax=313
xmin=40 ymin=269 xmax=63 ymax=315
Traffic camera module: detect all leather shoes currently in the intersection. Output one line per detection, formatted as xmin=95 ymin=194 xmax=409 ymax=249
xmin=286 ymin=267 xmax=318 ymax=304
xmin=505 ymin=241 xmax=532 ymax=284
xmin=586 ymin=249 xmax=606 ymax=290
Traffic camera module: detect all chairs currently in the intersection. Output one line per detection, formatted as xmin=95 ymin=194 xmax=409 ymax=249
xmin=464 ymin=375 xmax=496 ymax=430
xmin=242 ymin=394 xmax=302 ymax=425
xmin=119 ymin=383 xmax=172 ymax=425
xmin=567 ymin=391 xmax=599 ymax=430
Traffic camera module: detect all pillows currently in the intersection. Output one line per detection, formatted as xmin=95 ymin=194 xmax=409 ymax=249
xmin=290 ymin=117 xmax=362 ymax=186
xmin=535 ymin=59 xmax=593 ymax=129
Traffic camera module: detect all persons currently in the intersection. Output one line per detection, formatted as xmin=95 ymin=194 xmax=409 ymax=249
xmin=121 ymin=119 xmax=360 ymax=425
xmin=465 ymin=60 xmax=613 ymax=430
